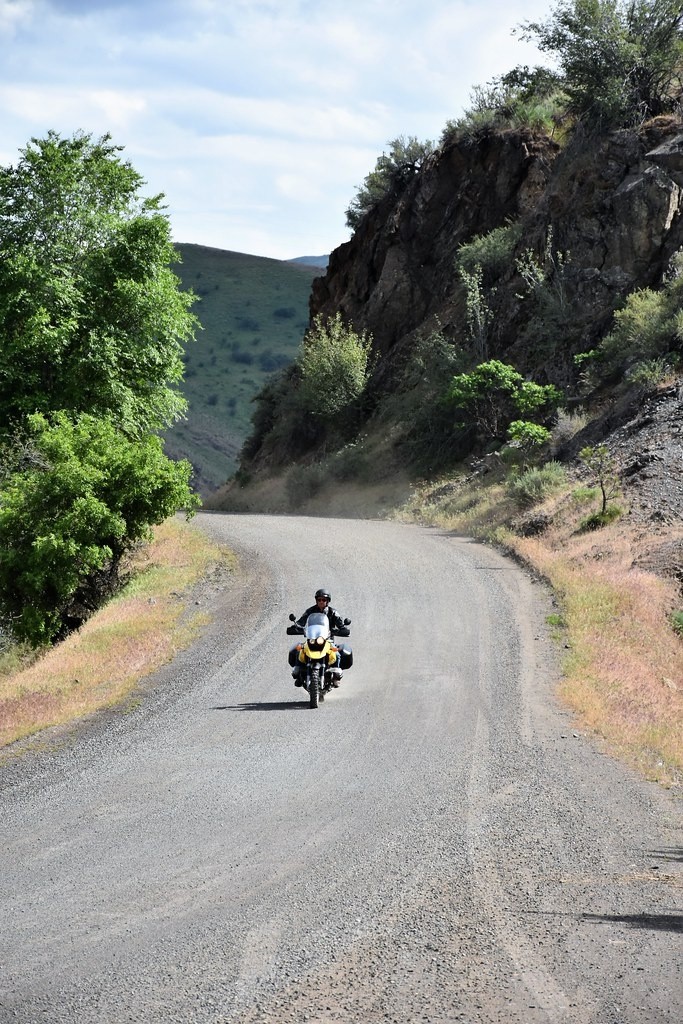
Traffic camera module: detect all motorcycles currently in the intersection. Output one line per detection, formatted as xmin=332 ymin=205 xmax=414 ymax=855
xmin=288 ymin=613 xmax=352 ymax=708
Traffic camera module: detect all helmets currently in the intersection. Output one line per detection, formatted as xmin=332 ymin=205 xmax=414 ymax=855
xmin=315 ymin=589 xmax=331 ymax=602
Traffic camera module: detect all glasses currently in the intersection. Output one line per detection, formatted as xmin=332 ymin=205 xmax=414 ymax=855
xmin=317 ymin=598 xmax=327 ymax=602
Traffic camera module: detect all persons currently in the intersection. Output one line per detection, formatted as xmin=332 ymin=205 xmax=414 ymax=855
xmin=287 ymin=589 xmax=350 ymax=687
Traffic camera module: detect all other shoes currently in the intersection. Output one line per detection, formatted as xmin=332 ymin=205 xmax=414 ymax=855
xmin=332 ymin=676 xmax=340 ymax=688
xmin=295 ymin=680 xmax=303 ymax=687
